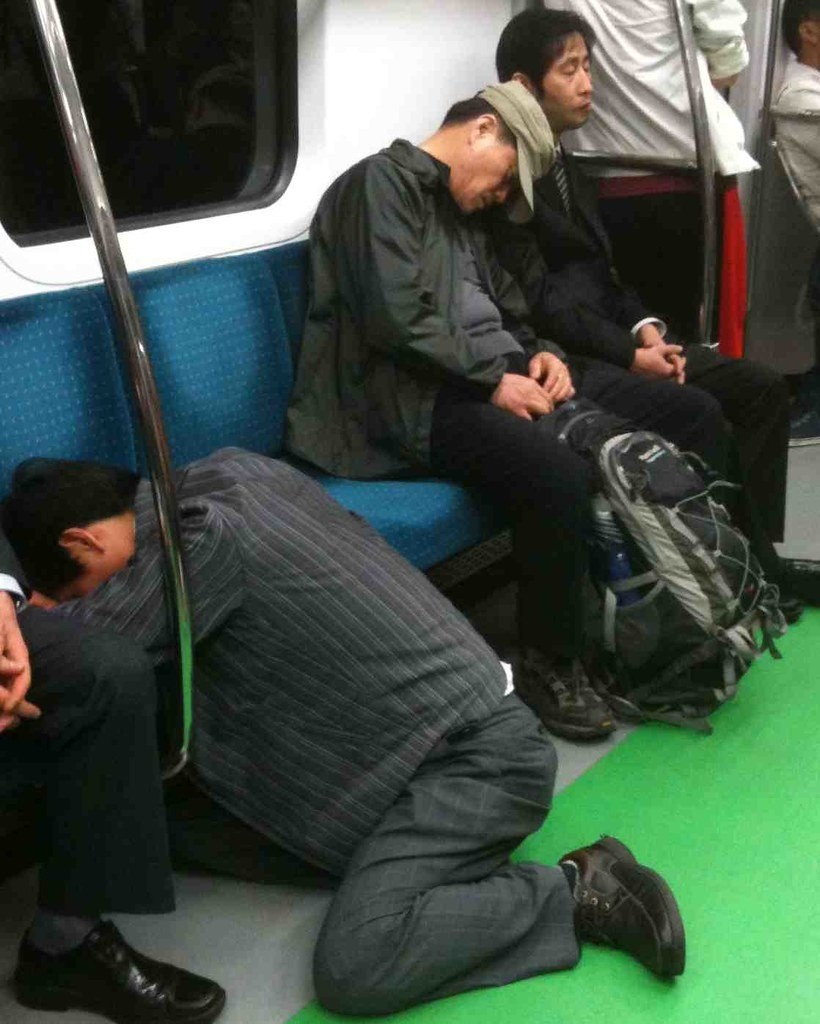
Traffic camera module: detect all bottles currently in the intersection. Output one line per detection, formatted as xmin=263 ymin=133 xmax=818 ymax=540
xmin=606 ymin=550 xmax=641 ymax=607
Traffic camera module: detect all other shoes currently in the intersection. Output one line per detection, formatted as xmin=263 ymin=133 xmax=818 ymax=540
xmin=752 ymin=539 xmax=812 ymax=624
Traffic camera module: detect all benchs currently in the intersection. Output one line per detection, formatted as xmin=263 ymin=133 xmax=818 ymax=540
xmin=0 ymin=236 xmax=515 ymax=575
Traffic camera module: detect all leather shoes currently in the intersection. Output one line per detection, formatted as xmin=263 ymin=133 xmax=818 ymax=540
xmin=562 ymin=838 xmax=684 ymax=979
xmin=9 ymin=918 xmax=225 ymax=1023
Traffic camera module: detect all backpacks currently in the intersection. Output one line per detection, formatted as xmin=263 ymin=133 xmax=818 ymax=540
xmin=564 ymin=407 xmax=784 ymax=735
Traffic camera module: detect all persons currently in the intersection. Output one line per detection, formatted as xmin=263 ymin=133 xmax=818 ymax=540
xmin=4 ymin=444 xmax=688 ymax=1016
xmin=287 ymin=0 xmax=819 ymax=736
xmin=0 ymin=512 xmax=228 ymax=1023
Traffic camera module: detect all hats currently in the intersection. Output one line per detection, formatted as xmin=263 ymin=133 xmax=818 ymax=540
xmin=481 ymin=82 xmax=555 ymax=223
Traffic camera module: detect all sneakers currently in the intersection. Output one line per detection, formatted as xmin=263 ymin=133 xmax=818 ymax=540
xmin=512 ymin=652 xmax=617 ymax=737
